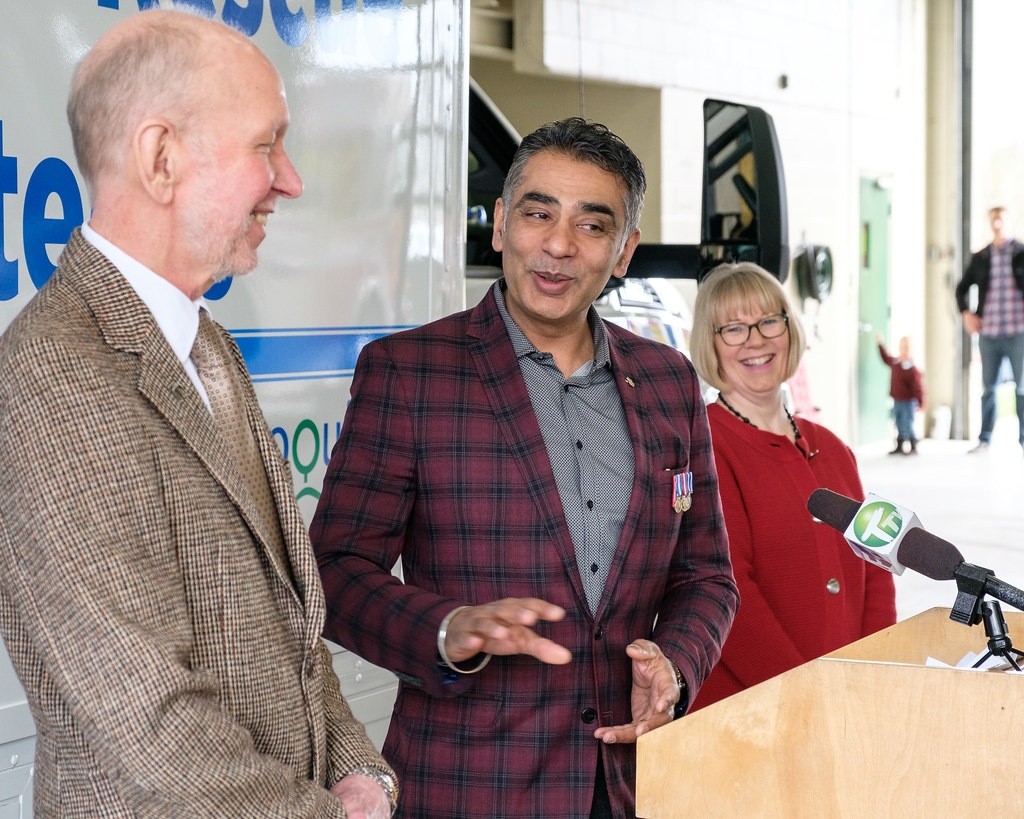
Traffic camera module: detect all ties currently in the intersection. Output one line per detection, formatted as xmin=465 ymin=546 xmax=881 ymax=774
xmin=190 ymin=307 xmax=291 ymax=580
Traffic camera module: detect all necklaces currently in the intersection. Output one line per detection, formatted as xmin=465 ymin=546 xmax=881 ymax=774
xmin=719 ymin=393 xmax=802 ymax=439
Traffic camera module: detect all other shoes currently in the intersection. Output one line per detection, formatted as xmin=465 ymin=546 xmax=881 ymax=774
xmin=967 ymin=440 xmax=990 ymax=455
xmin=907 ymin=449 xmax=918 ymax=456
xmin=888 ymin=449 xmax=904 ymax=456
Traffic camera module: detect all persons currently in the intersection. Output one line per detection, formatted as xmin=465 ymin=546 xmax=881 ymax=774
xmin=308 ymin=119 xmax=741 ymax=819
xmin=1 ymin=6 xmax=400 ymax=819
xmin=875 ymin=327 xmax=926 ymax=457
xmin=683 ymin=258 xmax=899 ymax=714
xmin=955 ymin=207 xmax=1024 ymax=454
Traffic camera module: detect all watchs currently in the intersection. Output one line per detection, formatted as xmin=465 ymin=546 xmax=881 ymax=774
xmin=351 ymin=768 xmax=398 ymax=819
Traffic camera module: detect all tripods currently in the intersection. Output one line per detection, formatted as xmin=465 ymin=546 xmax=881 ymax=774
xmin=971 ymin=600 xmax=1024 ymax=671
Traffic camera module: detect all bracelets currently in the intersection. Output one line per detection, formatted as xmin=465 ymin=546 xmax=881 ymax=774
xmin=674 ymin=662 xmax=686 ymax=713
xmin=438 ymin=606 xmax=492 ymax=674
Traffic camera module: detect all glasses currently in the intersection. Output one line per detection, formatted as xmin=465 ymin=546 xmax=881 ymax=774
xmin=713 ymin=313 xmax=789 ymax=346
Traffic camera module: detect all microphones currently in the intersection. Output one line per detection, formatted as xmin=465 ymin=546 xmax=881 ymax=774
xmin=807 ymin=488 xmax=1023 ymax=612
